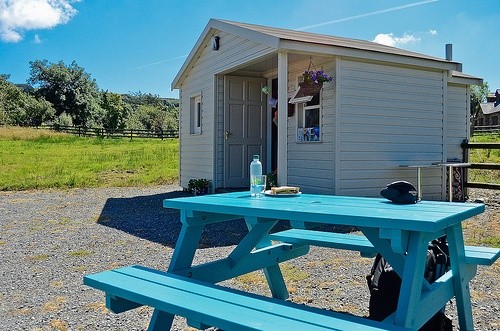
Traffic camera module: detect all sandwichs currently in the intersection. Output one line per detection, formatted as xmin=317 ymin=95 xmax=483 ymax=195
xmin=271 ymin=186 xmax=300 ymax=194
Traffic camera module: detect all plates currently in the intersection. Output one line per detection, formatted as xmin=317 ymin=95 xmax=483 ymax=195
xmin=264 ymin=190 xmax=302 ymax=195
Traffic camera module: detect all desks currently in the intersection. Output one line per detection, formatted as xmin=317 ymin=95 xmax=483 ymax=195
xmin=146 ymin=191 xmax=485 ymax=331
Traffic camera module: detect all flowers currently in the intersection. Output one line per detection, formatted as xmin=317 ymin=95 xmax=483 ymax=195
xmin=302 ymin=71 xmax=332 ymax=84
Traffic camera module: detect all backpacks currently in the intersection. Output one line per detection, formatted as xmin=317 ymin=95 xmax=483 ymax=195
xmin=368 ymin=237 xmax=452 ymax=331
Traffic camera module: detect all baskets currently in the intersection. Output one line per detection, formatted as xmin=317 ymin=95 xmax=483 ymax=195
xmin=299 ymin=82 xmax=323 ymax=96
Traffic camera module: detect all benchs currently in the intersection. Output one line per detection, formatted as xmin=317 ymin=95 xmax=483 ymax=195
xmin=268 ymin=229 xmax=500 ymax=266
xmin=83 ymin=263 xmax=419 ymax=331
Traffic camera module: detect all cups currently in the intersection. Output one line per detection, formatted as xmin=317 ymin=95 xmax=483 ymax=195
xmin=255 ymin=175 xmax=266 ymax=199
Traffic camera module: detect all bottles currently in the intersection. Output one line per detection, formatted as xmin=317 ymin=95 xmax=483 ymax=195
xmin=250 ymin=154 xmax=262 ymax=196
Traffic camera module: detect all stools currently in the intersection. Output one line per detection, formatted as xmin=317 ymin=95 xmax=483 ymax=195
xmin=398 ymin=162 xmax=471 ymax=202
